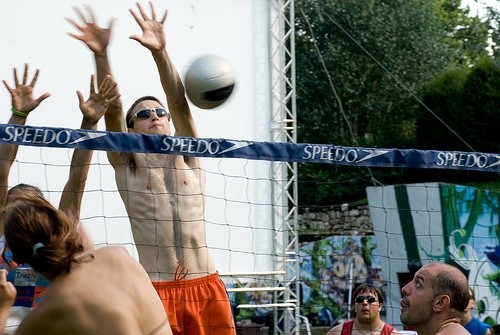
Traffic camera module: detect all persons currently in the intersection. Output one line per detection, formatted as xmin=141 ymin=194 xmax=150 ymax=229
xmin=1 ymin=63 xmax=173 ymax=335
xmin=326 ymin=283 xmax=401 ymax=335
xmin=460 ymin=287 xmax=493 ymax=335
xmin=66 ymin=0 xmax=236 ymax=335
xmin=400 ymin=262 xmax=471 ymax=335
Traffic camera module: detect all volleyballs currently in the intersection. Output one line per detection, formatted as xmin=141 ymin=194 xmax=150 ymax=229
xmin=184 ymin=55 xmax=235 ymax=109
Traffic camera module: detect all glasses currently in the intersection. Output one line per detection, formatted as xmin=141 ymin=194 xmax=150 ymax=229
xmin=355 ymin=295 xmax=380 ymax=304
xmin=129 ymin=108 xmax=171 ymax=124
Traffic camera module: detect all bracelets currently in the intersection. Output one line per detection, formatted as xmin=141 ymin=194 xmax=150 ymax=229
xmin=12 ymin=107 xmax=28 ymax=117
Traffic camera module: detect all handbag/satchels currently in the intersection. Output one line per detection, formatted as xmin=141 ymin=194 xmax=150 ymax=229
xmin=252 ymin=310 xmax=267 ymax=323
xmin=266 ymin=310 xmax=285 ymax=328
xmin=305 ymin=313 xmax=317 ymax=325
xmin=318 ymin=307 xmax=336 ymax=326
